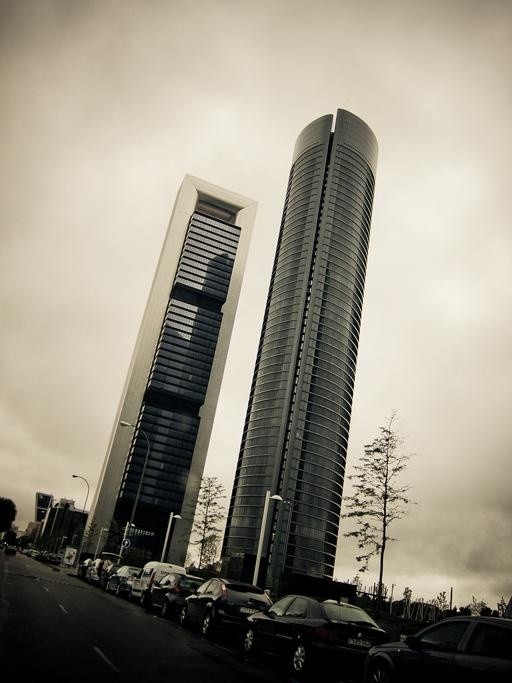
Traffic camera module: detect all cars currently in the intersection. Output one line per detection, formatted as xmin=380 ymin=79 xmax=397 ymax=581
xmin=365 ymin=614 xmax=511 ymax=683
xmin=241 ymin=595 xmax=393 ymax=677
xmin=76 ymin=558 xmax=276 ymax=646
xmin=1 ymin=544 xmax=62 ymax=566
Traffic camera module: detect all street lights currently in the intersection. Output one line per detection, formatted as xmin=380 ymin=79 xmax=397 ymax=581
xmin=40 ymin=535 xmax=78 ymax=553
xmin=160 ymin=512 xmax=182 ymax=564
xmin=93 ymin=526 xmax=110 ymax=562
xmin=118 ymin=521 xmax=136 ymax=566
xmin=251 ymin=490 xmax=283 ymax=588
xmin=113 ymin=417 xmax=150 ymax=566
xmin=69 ymin=474 xmax=90 ymax=554
